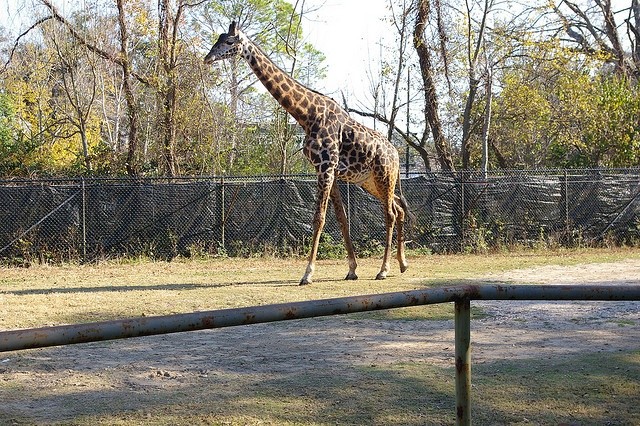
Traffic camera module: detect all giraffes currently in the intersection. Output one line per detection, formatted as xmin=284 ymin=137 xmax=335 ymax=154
xmin=204 ymin=21 xmax=417 ymax=285
